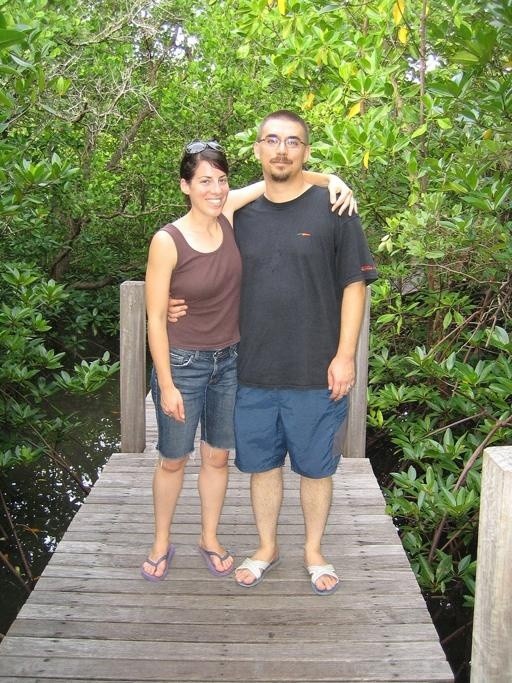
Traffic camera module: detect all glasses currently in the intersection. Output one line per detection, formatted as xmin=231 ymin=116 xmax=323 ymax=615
xmin=186 ymin=141 xmax=225 ymax=154
xmin=257 ymin=136 xmax=306 ymax=149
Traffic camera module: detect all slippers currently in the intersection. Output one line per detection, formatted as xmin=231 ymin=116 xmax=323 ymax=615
xmin=140 ymin=542 xmax=174 ymax=583
xmin=198 ymin=542 xmax=234 ymax=578
xmin=303 ymin=552 xmax=340 ymax=597
xmin=235 ymin=549 xmax=281 ymax=588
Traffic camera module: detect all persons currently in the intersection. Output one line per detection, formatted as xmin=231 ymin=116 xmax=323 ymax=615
xmin=167 ymin=108 xmax=379 ymax=595
xmin=140 ymin=140 xmax=362 ymax=579
